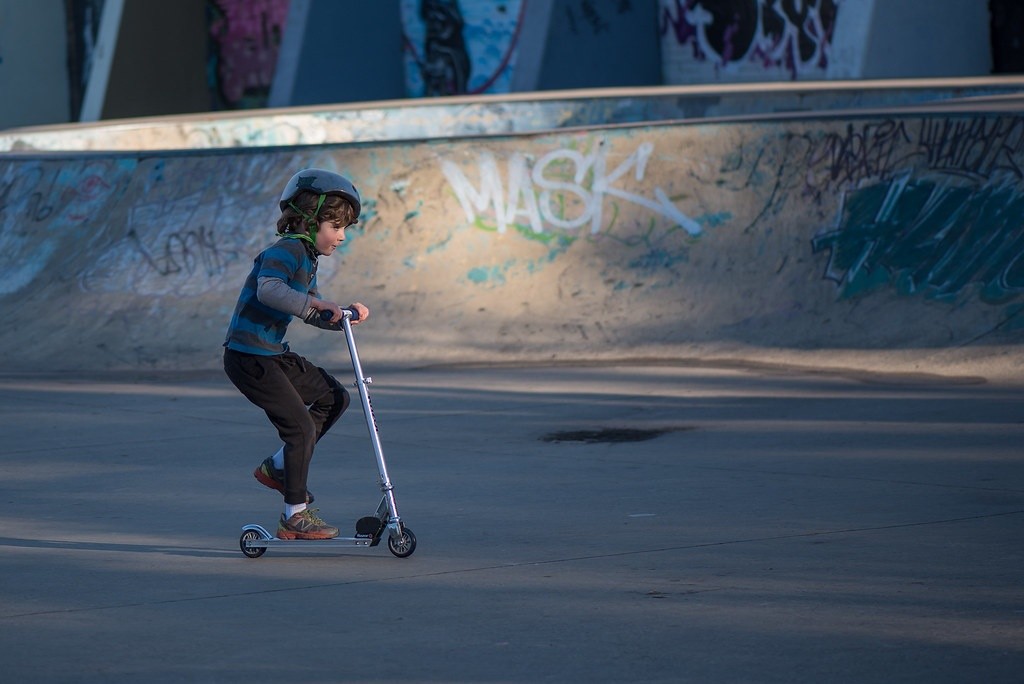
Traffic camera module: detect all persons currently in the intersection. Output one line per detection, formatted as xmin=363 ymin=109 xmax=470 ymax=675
xmin=223 ymin=169 xmax=369 ymax=540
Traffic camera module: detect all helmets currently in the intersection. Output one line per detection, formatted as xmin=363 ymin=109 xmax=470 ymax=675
xmin=279 ymin=167 xmax=361 ymax=229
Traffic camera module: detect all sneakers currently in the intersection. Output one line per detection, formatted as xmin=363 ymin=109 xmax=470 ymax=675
xmin=254 ymin=456 xmax=314 ymax=505
xmin=276 ymin=507 xmax=339 ymax=540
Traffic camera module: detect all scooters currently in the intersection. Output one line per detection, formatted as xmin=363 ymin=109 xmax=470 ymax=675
xmin=240 ymin=308 xmax=417 ymax=558
